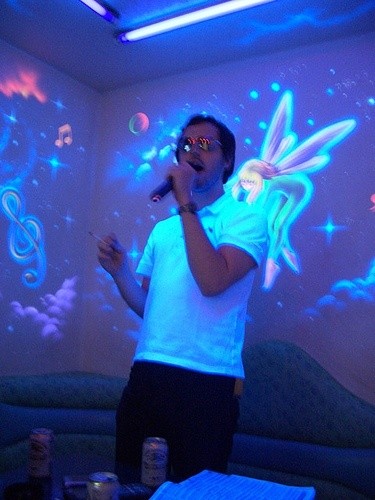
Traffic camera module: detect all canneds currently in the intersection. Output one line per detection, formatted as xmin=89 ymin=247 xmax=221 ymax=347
xmin=29 ymin=428 xmax=53 ymax=478
xmin=142 ymin=437 xmax=168 ymax=487
xmin=86 ymin=472 xmax=120 ymax=500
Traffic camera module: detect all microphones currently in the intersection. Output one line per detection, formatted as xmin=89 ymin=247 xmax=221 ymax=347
xmin=150 ymin=178 xmax=173 ymax=203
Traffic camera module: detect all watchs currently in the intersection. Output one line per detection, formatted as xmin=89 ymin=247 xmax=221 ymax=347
xmin=177 ymin=201 xmax=198 ymax=214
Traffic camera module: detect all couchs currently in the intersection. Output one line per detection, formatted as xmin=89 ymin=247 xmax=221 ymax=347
xmin=0 ymin=341 xmax=375 ymax=500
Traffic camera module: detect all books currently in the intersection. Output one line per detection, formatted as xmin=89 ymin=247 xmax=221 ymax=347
xmin=149 ymin=470 xmax=316 ymax=500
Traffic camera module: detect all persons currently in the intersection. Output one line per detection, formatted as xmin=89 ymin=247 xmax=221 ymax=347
xmin=97 ymin=114 xmax=268 ymax=488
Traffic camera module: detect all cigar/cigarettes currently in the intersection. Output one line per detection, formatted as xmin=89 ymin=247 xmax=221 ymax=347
xmin=89 ymin=232 xmax=105 ymax=244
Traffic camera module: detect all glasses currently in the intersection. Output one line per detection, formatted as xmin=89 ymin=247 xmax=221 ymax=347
xmin=177 ymin=136 xmax=223 ymax=153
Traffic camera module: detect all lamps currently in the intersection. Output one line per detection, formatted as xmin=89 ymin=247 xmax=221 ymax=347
xmin=120 ymin=0 xmax=274 ymax=43
xmin=80 ymin=0 xmax=115 ymax=23
